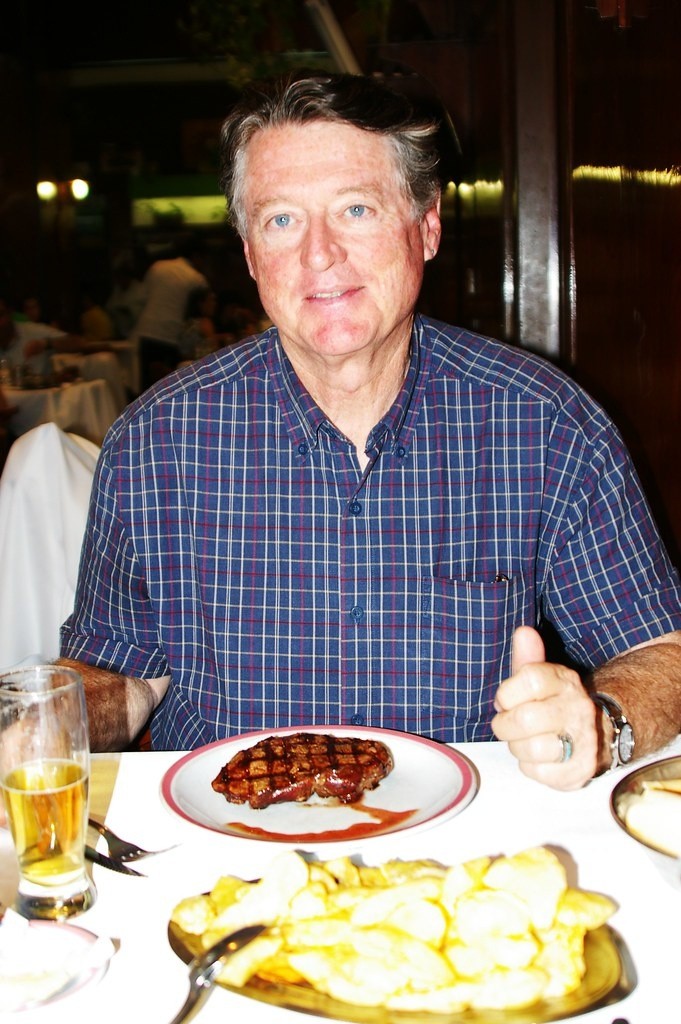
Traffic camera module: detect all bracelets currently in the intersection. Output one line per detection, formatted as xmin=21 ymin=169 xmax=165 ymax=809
xmin=44 ymin=337 xmax=52 ymax=350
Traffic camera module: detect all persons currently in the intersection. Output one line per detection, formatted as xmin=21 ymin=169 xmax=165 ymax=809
xmin=0 ymin=73 xmax=681 ymax=792
xmin=0 ymin=235 xmax=261 ymax=393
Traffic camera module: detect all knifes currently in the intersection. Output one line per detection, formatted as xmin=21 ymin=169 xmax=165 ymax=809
xmin=83 ymin=844 xmax=148 ymax=878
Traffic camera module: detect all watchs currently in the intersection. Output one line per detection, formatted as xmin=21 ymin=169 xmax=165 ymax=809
xmin=590 ymin=693 xmax=635 ymax=770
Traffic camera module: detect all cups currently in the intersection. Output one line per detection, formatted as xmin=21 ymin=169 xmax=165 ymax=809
xmin=0 ymin=665 xmax=97 ymax=921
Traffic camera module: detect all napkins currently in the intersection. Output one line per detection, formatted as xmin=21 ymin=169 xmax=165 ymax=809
xmin=0 ymin=906 xmax=117 ymax=1019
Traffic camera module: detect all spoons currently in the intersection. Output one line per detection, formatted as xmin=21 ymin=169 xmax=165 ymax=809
xmin=170 ymin=925 xmax=267 ymax=1024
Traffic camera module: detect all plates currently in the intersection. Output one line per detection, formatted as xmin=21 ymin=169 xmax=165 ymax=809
xmin=609 ymin=755 xmax=681 ymax=862
xmin=160 ymin=726 xmax=477 ymax=847
xmin=168 ymin=876 xmax=623 ymax=1023
xmin=0 ymin=919 xmax=110 ymax=1013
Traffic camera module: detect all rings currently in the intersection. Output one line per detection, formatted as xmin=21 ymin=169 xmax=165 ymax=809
xmin=558 ymin=732 xmax=572 ymax=763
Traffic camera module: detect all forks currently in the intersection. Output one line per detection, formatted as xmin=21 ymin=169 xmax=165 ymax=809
xmin=88 ymin=817 xmax=177 ymax=864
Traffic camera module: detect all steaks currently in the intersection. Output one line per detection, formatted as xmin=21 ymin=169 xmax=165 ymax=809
xmin=212 ymin=732 xmax=393 ymax=808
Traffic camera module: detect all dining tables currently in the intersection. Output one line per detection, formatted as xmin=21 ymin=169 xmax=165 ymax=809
xmin=0 ymin=738 xmax=681 ymax=1024
